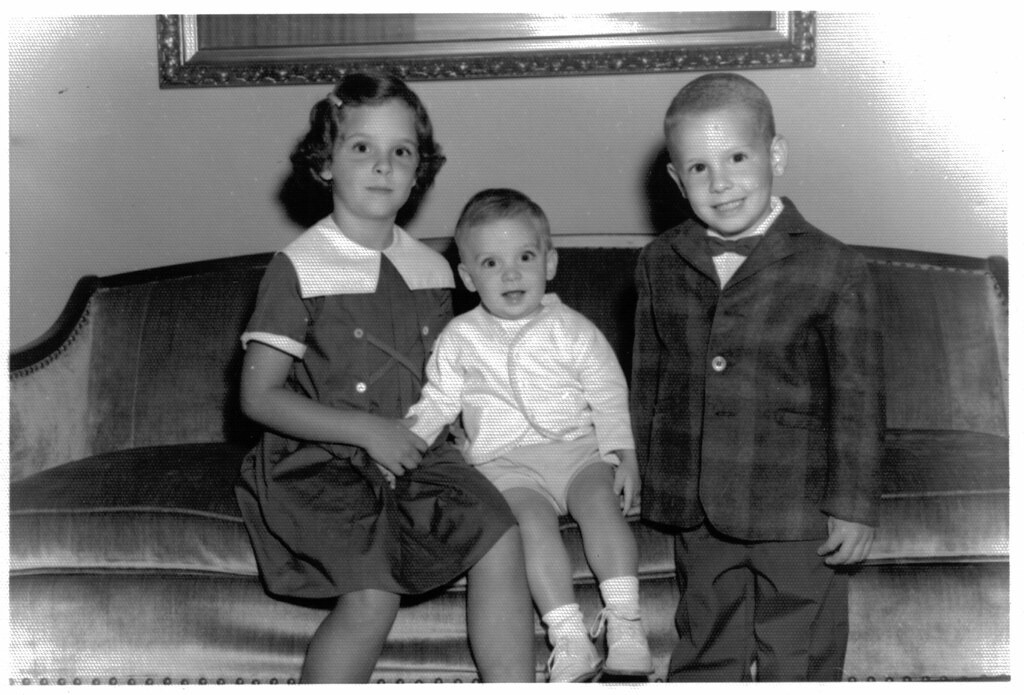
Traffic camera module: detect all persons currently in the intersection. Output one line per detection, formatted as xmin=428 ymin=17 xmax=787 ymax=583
xmin=237 ymin=72 xmax=537 ymax=682
xmin=632 ymin=65 xmax=885 ymax=681
xmin=376 ymin=185 xmax=655 ymax=684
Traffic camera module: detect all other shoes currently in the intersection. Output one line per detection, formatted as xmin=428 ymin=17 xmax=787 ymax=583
xmin=590 ymin=608 xmax=652 ymax=675
xmin=547 ymin=631 xmax=601 ymax=683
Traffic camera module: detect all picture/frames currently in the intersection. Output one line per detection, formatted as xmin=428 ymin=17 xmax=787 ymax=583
xmin=155 ymin=9 xmax=818 ymax=89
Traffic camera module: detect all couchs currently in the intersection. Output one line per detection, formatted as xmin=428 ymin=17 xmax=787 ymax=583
xmin=10 ymin=232 xmax=1009 ymax=685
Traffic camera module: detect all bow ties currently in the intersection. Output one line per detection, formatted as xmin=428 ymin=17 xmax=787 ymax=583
xmin=702 ymin=234 xmax=764 ymax=258
